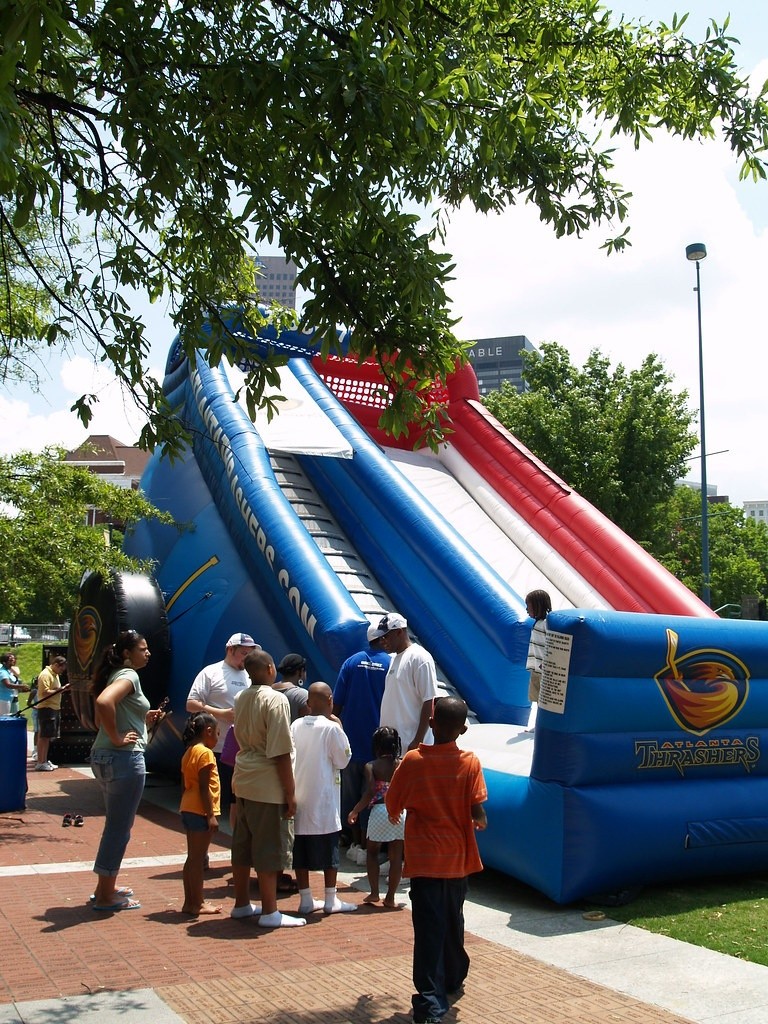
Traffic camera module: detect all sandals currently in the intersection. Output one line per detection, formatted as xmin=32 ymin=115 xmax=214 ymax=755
xmin=276 ymin=873 xmax=298 ymax=890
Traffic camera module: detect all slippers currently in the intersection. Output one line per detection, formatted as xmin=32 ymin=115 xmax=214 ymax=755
xmin=91 ymin=898 xmax=141 ymax=910
xmin=90 ymin=887 xmax=133 ymax=900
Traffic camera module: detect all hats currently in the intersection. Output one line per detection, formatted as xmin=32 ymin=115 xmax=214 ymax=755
xmin=226 ymin=633 xmax=262 ymax=650
xmin=374 ymin=612 xmax=407 ymax=637
xmin=367 ymin=621 xmax=381 ymax=642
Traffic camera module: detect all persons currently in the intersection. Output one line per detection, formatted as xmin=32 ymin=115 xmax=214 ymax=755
xmin=90 ymin=629 xmax=161 ymax=910
xmin=36 ymin=657 xmax=71 ymax=770
xmin=230 ymin=650 xmax=306 ymax=926
xmin=27 ymin=676 xmax=39 ymax=758
xmin=289 ymin=682 xmax=358 ymax=914
xmin=186 ymin=635 xmax=311 ymax=891
xmin=385 ymin=697 xmax=488 ymax=1024
xmin=524 ymin=589 xmax=553 ymax=731
xmin=374 ymin=613 xmax=437 ymax=884
xmin=332 ymin=623 xmax=391 ymax=866
xmin=0 ymin=653 xmax=30 ymax=714
xmin=347 ymin=726 xmax=402 ymax=906
xmin=180 ymin=714 xmax=223 ymax=914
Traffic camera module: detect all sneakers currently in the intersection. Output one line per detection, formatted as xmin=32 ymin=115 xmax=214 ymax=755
xmin=35 ymin=762 xmax=54 ymax=771
xmin=379 ymin=860 xmax=405 ymax=876
xmin=356 ymin=848 xmax=387 ymax=866
xmin=346 ymin=844 xmax=362 ymax=861
xmin=75 ymin=815 xmax=84 ymax=826
xmin=48 ymin=761 xmax=58 ymax=769
xmin=386 ymin=874 xmax=411 ymax=884
xmin=62 ymin=813 xmax=72 ymax=825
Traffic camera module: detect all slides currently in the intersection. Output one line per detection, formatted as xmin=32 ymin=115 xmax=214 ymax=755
xmin=174 ymin=311 xmax=724 ymax=725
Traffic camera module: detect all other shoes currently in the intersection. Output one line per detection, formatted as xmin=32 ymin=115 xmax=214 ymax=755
xmin=32 ymin=749 xmax=40 ymax=761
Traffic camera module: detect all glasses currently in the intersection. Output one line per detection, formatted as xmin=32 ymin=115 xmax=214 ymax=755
xmin=431 ymin=697 xmax=466 ymax=719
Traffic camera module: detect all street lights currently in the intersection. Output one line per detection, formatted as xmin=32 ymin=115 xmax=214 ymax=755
xmin=684 ymin=242 xmax=710 ymax=609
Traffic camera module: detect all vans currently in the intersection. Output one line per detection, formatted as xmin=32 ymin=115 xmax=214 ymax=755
xmin=0 ymin=623 xmax=32 ymax=645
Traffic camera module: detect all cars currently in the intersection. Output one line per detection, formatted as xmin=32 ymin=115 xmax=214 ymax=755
xmin=40 ymin=634 xmax=59 ymax=642
